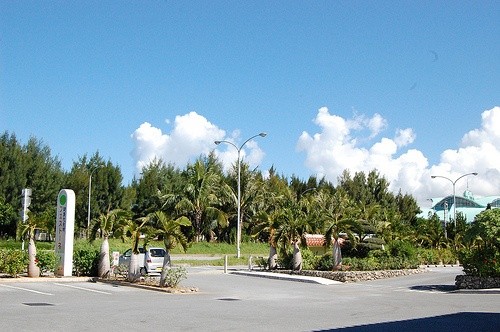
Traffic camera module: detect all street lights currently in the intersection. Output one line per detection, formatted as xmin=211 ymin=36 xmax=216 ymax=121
xmin=88 ymin=165 xmax=104 ymax=239
xmin=215 ymin=132 xmax=266 ymax=258
xmin=432 ymin=171 xmax=478 ymax=229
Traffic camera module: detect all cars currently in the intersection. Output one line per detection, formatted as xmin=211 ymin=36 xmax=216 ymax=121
xmin=28 ymin=228 xmax=53 ymax=242
xmin=118 ymin=247 xmax=171 ymax=274
